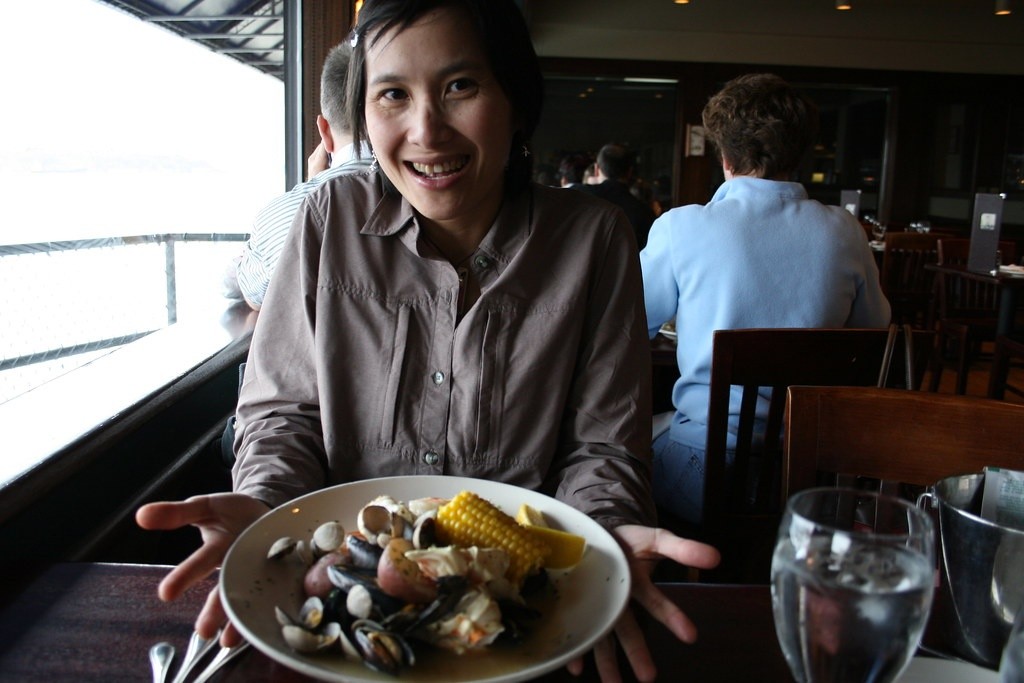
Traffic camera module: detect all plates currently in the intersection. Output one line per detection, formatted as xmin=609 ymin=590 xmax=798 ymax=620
xmin=1000 ymin=265 xmax=1024 ymax=275
xmin=657 ymin=314 xmax=677 ymax=340
xmin=218 ymin=474 xmax=631 ymax=683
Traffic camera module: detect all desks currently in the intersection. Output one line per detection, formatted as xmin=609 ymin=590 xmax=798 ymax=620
xmin=0 ymin=559 xmax=957 ymax=683
xmin=923 ymin=263 xmax=1024 ymax=402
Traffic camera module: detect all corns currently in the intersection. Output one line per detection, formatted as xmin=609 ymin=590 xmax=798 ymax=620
xmin=435 ymin=490 xmax=552 ymax=582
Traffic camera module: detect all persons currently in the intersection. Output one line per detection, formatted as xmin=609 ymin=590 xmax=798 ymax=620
xmin=135 ymin=0 xmax=721 ymax=683
xmin=640 ymin=76 xmax=891 ymax=518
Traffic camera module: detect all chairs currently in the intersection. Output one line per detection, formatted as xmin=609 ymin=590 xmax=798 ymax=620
xmin=864 ymin=223 xmax=1023 ymax=402
xmin=780 ymin=385 xmax=1023 ymax=575
xmin=700 ymin=328 xmax=937 ymax=584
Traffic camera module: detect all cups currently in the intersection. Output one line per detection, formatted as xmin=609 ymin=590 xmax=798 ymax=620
xmin=916 ymin=474 xmax=1024 ymax=671
xmin=769 ymin=488 xmax=936 ymax=682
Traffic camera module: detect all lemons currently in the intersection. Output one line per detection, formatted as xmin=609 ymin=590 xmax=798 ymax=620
xmin=517 ymin=504 xmax=587 ymax=570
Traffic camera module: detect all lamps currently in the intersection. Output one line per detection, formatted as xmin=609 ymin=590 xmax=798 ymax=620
xmin=835 ymin=0 xmax=851 ymax=10
xmin=994 ymin=0 xmax=1013 ymax=15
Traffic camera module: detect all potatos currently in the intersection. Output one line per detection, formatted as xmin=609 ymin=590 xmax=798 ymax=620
xmin=305 ymin=552 xmax=351 ymax=598
xmin=377 ymin=536 xmax=441 ymax=600
